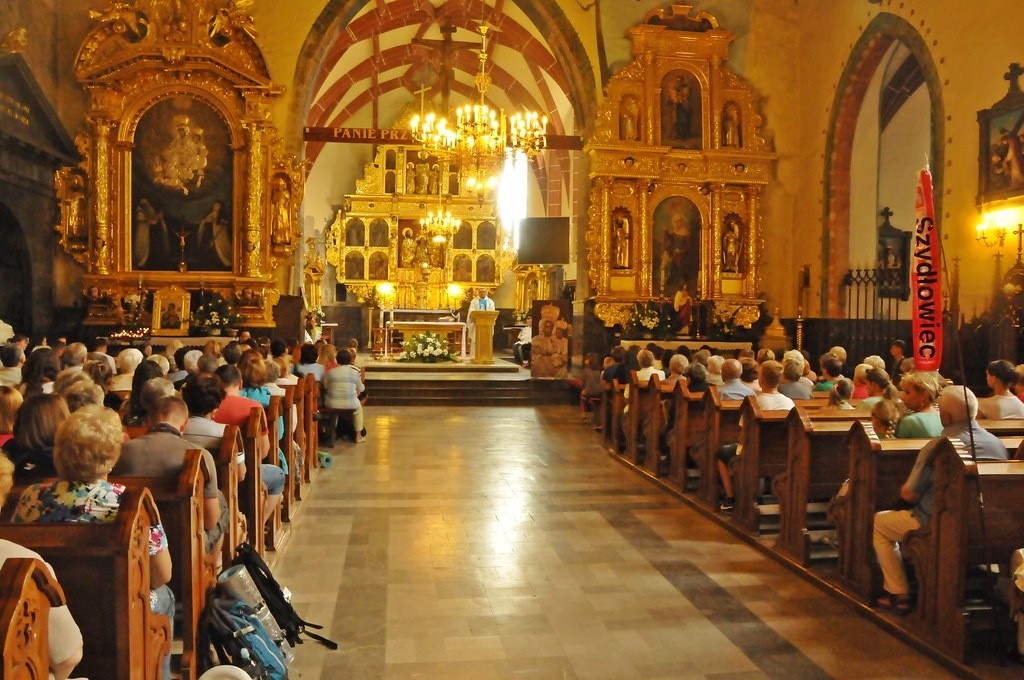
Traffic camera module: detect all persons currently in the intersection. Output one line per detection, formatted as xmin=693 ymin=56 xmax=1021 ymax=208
xmin=514 ymin=315 xmax=532 ymax=367
xmin=273 ymin=178 xmax=290 ymax=244
xmin=674 ymin=283 xmax=693 ymax=334
xmin=0 ymin=333 xmax=369 ymax=680
xmin=612 ymin=218 xmax=629 ymax=267
xmin=406 ymin=165 xmax=439 ymax=194
xmin=401 ymin=229 xmax=439 ymax=268
xmin=467 ymin=289 xmax=495 ymax=359
xmin=562 ymin=340 xmax=1024 ymax=663
xmin=723 ymin=221 xmax=741 ymax=272
xmin=160 ymin=304 xmax=180 ymax=327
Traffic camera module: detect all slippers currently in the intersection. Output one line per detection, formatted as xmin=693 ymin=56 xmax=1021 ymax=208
xmin=355 ymin=436 xmax=365 ymax=442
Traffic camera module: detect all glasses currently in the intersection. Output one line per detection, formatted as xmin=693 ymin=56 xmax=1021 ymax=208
xmin=480 ymin=291 xmax=485 ymax=293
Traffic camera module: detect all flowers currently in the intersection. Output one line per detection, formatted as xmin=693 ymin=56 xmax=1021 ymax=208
xmin=397 ymin=330 xmax=462 ymax=363
xmin=627 ymin=299 xmax=671 ymax=336
xmin=191 ymin=297 xmax=251 ymax=333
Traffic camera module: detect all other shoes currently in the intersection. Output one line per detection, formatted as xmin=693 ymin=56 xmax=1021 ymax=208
xmin=593 ymin=425 xmax=602 ymax=432
xmin=721 ymin=499 xmax=735 ymax=510
xmin=820 ymin=534 xmax=840 ymax=547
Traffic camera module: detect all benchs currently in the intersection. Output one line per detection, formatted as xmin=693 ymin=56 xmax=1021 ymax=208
xmin=589 ymin=368 xmax=1024 ymax=666
xmin=0 ymin=372 xmax=353 ymax=680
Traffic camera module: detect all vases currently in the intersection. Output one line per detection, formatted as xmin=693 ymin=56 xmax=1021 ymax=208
xmin=422 ymin=356 xmax=437 ymax=363
xmin=208 ymin=328 xmax=221 ymax=335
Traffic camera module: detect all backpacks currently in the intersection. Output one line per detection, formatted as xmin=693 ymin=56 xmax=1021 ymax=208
xmin=233 ymin=542 xmax=304 ymax=647
xmin=198 ymin=585 xmax=287 ymax=680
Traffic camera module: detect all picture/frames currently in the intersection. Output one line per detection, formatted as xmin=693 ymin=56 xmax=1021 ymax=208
xmin=150 ymin=284 xmax=191 ymax=336
xmin=975 ymin=62 xmax=1024 ymax=206
xmin=877 ymin=207 xmax=912 ymax=301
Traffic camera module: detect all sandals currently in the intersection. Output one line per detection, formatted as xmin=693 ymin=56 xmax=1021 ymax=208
xmin=871 ymin=592 xmax=912 ymax=615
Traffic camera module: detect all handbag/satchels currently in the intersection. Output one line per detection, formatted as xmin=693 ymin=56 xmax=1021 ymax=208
xmin=337 ymin=415 xmax=366 ymax=439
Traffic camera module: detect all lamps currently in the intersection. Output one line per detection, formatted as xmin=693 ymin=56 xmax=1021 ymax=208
xmin=409 ymin=0 xmax=552 ymax=284
xmin=976 ymin=210 xmax=1007 ymax=248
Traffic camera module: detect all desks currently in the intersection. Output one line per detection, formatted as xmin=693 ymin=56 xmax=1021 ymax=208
xmin=96 ymin=337 xmax=240 ymax=355
